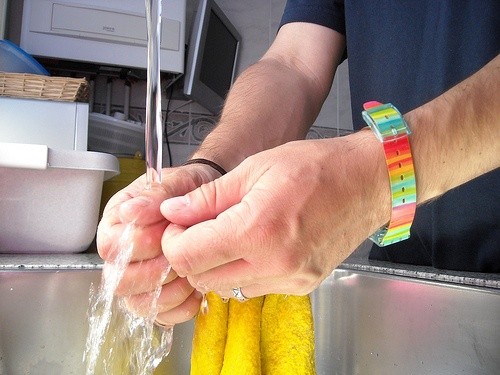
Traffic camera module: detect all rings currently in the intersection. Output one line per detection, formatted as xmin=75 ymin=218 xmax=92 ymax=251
xmin=151 ymin=318 xmax=175 ymax=330
xmin=232 ymin=286 xmax=250 ymax=304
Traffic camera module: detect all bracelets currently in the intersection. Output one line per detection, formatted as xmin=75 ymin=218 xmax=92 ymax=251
xmin=359 ymin=99 xmax=417 ymax=248
xmin=179 ymin=159 xmax=230 ymax=176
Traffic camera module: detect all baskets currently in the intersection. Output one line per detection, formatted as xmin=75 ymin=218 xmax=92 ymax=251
xmin=0 ymin=70 xmax=88 ymax=102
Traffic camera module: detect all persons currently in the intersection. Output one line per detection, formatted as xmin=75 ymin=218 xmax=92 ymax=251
xmin=89 ymin=0 xmax=500 ymax=331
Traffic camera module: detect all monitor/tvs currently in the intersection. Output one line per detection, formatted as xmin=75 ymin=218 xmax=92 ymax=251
xmin=184 ymin=1 xmax=242 ymax=116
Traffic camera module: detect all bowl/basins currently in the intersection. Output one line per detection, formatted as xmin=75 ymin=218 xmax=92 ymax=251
xmin=2 ymin=38 xmax=48 ymax=77
